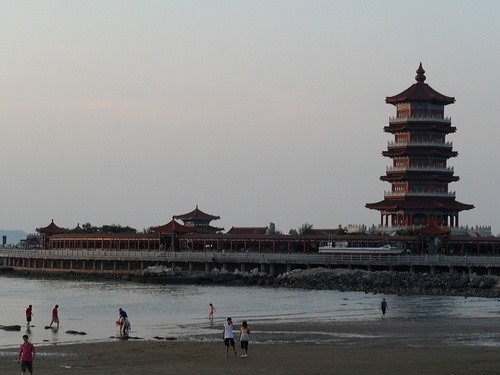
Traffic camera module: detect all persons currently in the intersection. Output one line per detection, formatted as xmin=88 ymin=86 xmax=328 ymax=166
xmin=239 ymin=321 xmax=250 ymax=358
xmin=50 ymin=304 xmax=59 ymax=331
xmin=115 ymin=308 xmax=132 ymax=340
xmin=381 ymin=298 xmax=389 ymax=320
xmin=26 ymin=304 xmax=34 ymax=325
xmin=17 ymin=335 xmax=36 ymax=375
xmin=208 ymin=303 xmax=216 ymax=321
xmin=407 ymin=248 xmax=411 ymax=256
xmin=223 ymin=317 xmax=236 ymax=356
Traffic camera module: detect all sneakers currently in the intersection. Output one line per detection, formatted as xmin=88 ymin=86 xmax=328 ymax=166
xmin=241 ymin=354 xmax=247 ymax=358
